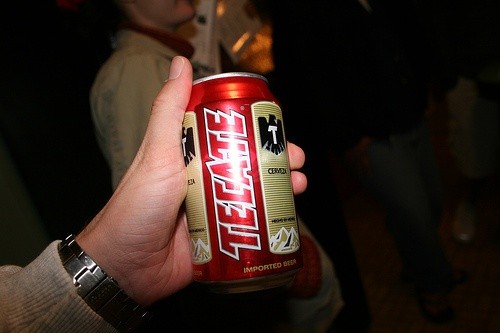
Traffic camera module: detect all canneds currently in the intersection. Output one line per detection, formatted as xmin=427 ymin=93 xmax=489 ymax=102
xmin=180 ymin=70 xmax=303 ymax=295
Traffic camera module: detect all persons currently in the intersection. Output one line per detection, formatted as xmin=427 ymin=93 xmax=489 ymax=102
xmin=91 ymin=0 xmax=356 ymax=333
xmin=0 ymin=54 xmax=306 ymax=333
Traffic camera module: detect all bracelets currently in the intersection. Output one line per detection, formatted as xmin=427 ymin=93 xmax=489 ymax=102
xmin=56 ymin=234 xmax=156 ymax=332
